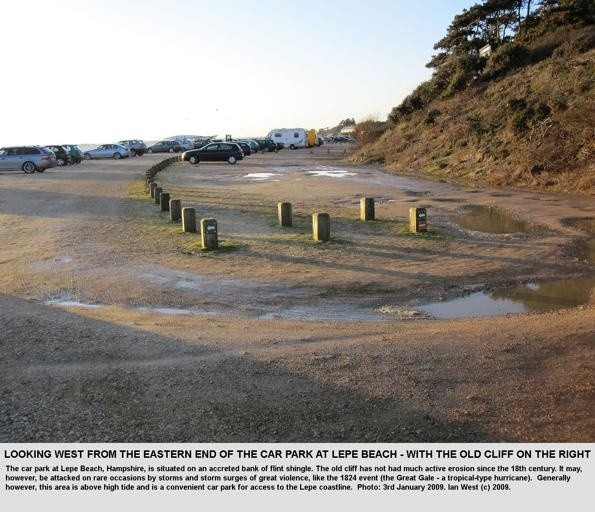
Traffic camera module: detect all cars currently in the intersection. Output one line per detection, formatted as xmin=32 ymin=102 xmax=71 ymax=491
xmin=324 ymin=136 xmax=353 ymax=142
xmin=210 ymin=139 xmax=224 ymax=142
xmin=83 ymin=144 xmax=130 ymax=160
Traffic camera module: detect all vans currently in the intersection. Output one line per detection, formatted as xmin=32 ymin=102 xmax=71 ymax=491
xmin=181 ymin=139 xmax=194 ymax=151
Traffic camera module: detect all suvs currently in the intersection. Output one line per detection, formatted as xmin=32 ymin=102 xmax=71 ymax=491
xmin=193 ymin=138 xmax=210 ymax=149
xmin=256 ymin=139 xmax=277 ymax=152
xmin=0 ymin=146 xmax=57 ymax=173
xmin=117 ymin=140 xmax=146 ymax=157
xmin=147 ymin=141 xmax=180 ymax=153
xmin=43 ymin=145 xmax=69 ymax=167
xmin=61 ymin=145 xmax=85 ymax=164
xmin=181 ymin=143 xmax=244 ymax=165
xmin=244 ymin=140 xmax=259 ymax=153
xmin=226 ymin=142 xmax=251 ymax=156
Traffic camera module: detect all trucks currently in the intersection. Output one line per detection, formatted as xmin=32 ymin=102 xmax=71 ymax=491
xmin=317 ymin=135 xmax=325 ymax=146
xmin=305 ymin=129 xmax=318 ymax=148
xmin=264 ymin=128 xmax=309 ymax=149
